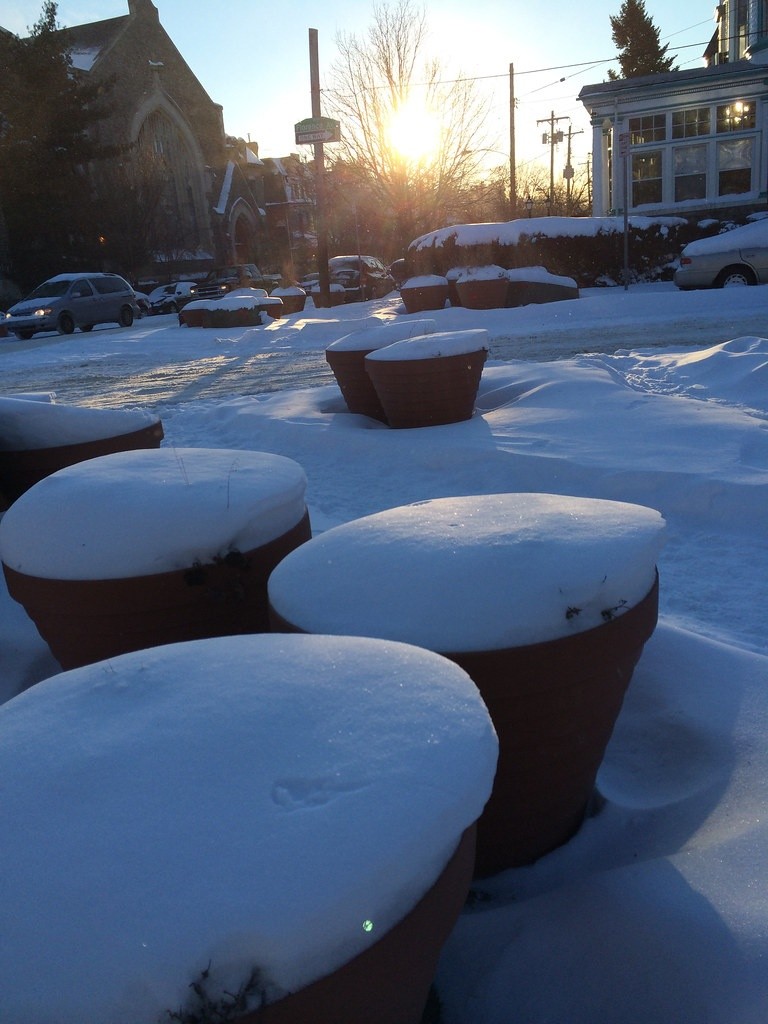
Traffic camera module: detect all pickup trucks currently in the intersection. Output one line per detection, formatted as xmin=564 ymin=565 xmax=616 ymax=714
xmin=190 ymin=264 xmax=279 ymax=300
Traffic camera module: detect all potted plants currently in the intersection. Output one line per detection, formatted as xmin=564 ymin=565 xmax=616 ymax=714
xmin=400 ymin=267 xmax=578 ymax=315
xmin=436 ymin=568 xmax=660 ymax=877
xmin=178 ymin=287 xmax=308 ymax=328
xmin=365 ymin=335 xmax=490 ymax=428
xmin=0 ymin=420 xmax=165 ymax=514
xmin=2 ymin=490 xmax=311 ymax=673
xmin=311 ymin=289 xmax=346 ymax=309
xmin=171 ymin=813 xmax=478 ymax=1024
xmin=325 ymin=350 xmax=387 ymax=423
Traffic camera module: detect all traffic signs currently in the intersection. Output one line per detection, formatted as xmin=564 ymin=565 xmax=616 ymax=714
xmin=294 ymin=116 xmax=341 ymax=145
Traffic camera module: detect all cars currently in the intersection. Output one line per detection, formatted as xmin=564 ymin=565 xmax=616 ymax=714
xmin=388 ymin=259 xmax=414 ymax=288
xmin=134 ymin=289 xmax=150 ymax=320
xmin=300 ymin=272 xmax=321 ymax=295
xmin=148 ymin=282 xmax=199 ymax=315
xmin=2 ymin=272 xmax=137 ymax=339
xmin=0 ymin=311 xmax=6 ymax=325
xmin=673 ymin=217 xmax=768 ymax=287
xmin=327 ymin=254 xmax=396 ymax=302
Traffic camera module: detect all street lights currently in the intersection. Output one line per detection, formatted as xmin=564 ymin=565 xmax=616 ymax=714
xmin=524 ymin=195 xmax=535 ymax=219
xmin=461 ymin=147 xmax=517 ymax=221
xmin=544 ymin=194 xmax=552 ymax=216
